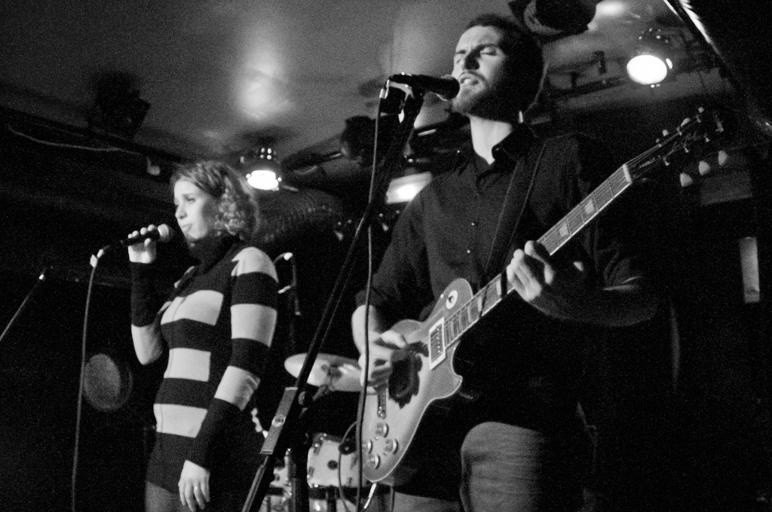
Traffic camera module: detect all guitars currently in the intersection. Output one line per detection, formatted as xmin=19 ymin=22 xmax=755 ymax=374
xmin=357 ymin=106 xmax=729 ymax=485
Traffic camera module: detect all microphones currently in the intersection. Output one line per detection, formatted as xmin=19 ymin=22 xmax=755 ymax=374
xmin=98 ymin=225 xmax=175 ymax=257
xmin=391 ymin=71 xmax=460 ymax=102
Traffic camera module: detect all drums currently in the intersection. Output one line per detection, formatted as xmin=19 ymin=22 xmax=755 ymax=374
xmin=307 ymin=434 xmax=358 ymax=511
xmin=263 ymin=451 xmax=297 ymax=511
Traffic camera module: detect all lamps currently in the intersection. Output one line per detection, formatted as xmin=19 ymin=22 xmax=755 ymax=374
xmin=621 ymin=33 xmax=678 ymax=86
xmin=244 ymin=134 xmax=283 ymax=193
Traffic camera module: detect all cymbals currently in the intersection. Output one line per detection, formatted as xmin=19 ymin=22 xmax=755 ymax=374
xmin=285 ymin=353 xmax=361 ymax=392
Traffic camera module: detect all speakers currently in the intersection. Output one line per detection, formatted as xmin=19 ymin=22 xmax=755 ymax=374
xmin=86 ymin=89 xmax=152 ymax=137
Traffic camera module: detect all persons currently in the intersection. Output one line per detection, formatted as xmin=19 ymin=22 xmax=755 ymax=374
xmin=126 ymin=159 xmax=278 ymax=512
xmin=351 ymin=13 xmax=657 ymax=512
